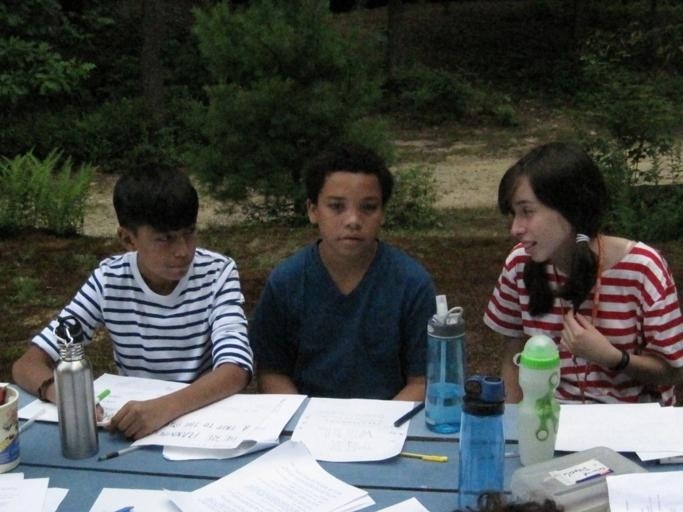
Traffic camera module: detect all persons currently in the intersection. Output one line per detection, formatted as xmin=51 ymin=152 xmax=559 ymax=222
xmin=482 ymin=140 xmax=683 ymax=405
xmin=12 ymin=163 xmax=254 ymax=441
xmin=249 ymin=140 xmax=437 ymax=402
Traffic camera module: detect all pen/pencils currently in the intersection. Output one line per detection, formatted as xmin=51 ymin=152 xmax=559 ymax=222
xmin=96 ymin=446 xmax=145 ymax=461
xmin=398 ymin=450 xmax=449 ymax=462
xmin=0 ymin=386 xmax=8 ymax=401
xmin=394 ymin=402 xmax=425 ymax=426
xmin=96 ymin=389 xmax=112 ymax=405
xmin=20 ymin=416 xmax=35 ymax=436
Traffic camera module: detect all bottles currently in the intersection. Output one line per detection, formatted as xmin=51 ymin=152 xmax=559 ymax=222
xmin=53 ymin=316 xmax=99 ymax=459
xmin=459 ymin=375 xmax=505 ymax=509
xmin=425 ymin=294 xmax=466 ymax=436
xmin=513 ymin=334 xmax=561 ymax=467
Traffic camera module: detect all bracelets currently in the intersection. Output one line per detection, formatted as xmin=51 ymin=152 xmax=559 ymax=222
xmin=609 ymin=350 xmax=630 ymax=372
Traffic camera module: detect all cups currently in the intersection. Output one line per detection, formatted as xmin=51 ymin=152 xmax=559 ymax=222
xmin=0 ymin=387 xmax=21 ymax=472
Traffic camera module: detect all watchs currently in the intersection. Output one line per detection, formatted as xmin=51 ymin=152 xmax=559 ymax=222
xmin=37 ymin=375 xmax=55 ymax=403
